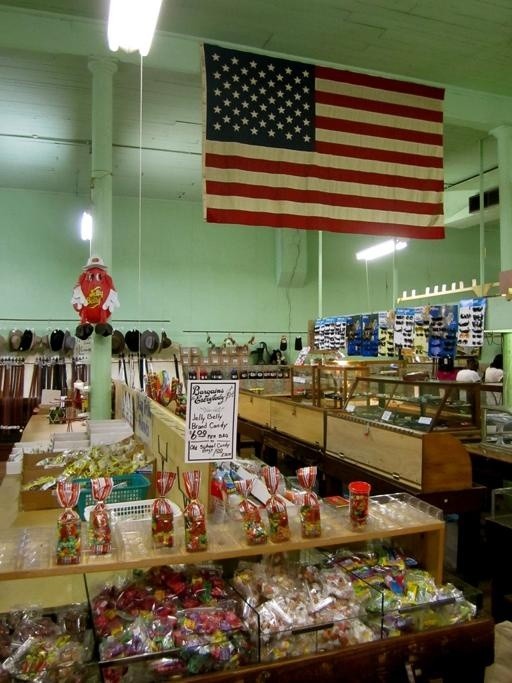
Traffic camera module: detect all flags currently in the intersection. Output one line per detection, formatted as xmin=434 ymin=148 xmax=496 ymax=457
xmin=199 ymin=41 xmax=447 ymax=245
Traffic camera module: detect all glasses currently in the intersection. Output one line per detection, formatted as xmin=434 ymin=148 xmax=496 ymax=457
xmin=346 ymin=321 xmax=394 ymax=356
xmin=394 ymin=314 xmax=457 ymax=357
xmin=458 ymin=339 xmax=468 ymax=344
xmin=458 ymin=321 xmax=469 ymax=326
xmin=459 ymin=329 xmax=470 ymax=333
xmin=472 ymin=334 xmax=482 ymax=338
xmin=472 ymin=341 xmax=481 ymax=345
xmin=472 ymin=321 xmax=483 ymax=327
xmin=472 ymin=329 xmax=482 ymax=334
xmin=313 ymin=322 xmax=346 ymax=351
xmin=460 ymin=313 xmax=471 ymax=318
xmin=472 ymin=306 xmax=483 ymax=312
xmin=473 ymin=314 xmax=483 ymax=320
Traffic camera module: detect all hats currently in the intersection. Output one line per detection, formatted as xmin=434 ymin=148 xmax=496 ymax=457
xmin=50 ymin=329 xmax=64 ymax=351
xmin=140 ymin=330 xmax=160 ymax=354
xmin=82 ymin=256 xmax=109 ymax=271
xmin=61 ymin=330 xmax=75 ymax=354
xmin=8 ymin=328 xmax=23 ymax=352
xmin=28 ymin=328 xmax=42 ymax=351
xmin=41 ymin=331 xmax=51 ymax=349
xmin=112 ymin=329 xmax=125 ymax=354
xmin=157 ymin=331 xmax=172 ymax=353
xmin=124 ymin=329 xmax=142 ymax=352
xmin=20 ymin=329 xmax=33 ymax=351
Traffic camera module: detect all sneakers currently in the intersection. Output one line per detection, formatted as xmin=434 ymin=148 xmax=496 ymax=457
xmin=94 ymin=322 xmax=114 ymax=337
xmin=74 ymin=322 xmax=93 ymax=341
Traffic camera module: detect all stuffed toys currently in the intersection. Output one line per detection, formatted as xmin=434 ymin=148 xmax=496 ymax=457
xmin=71 ymin=255 xmax=121 ymax=343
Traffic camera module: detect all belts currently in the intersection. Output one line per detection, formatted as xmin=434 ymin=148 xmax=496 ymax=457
xmin=71 ymin=357 xmax=88 ymax=392
xmin=118 ymin=351 xmax=154 ymax=393
xmin=26 ymin=357 xmax=67 ymax=422
xmin=0 ymin=357 xmax=25 ymax=426
xmin=174 ymin=355 xmax=180 ymax=382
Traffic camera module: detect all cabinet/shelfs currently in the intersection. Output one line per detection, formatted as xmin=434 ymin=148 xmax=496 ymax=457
xmin=0 ymin=490 xmax=494 ymax=683
xmin=0 ymin=378 xmax=216 ymax=525
xmin=270 ymin=363 xmax=371 ymax=450
xmin=181 ymin=347 xmax=293 ymax=397
xmin=239 ymin=377 xmax=320 ymax=430
xmin=325 ymin=375 xmax=482 ymax=495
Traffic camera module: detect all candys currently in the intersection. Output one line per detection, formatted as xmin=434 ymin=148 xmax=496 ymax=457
xmin=87 ymin=477 xmax=114 ymax=553
xmin=262 ymin=467 xmax=289 ymax=541
xmin=151 ymin=471 xmax=177 ymax=548
xmin=322 ymin=544 xmax=475 ymax=636
xmin=348 ymin=480 xmax=371 ymax=522
xmin=181 ymin=470 xmax=208 ymax=551
xmin=55 ymin=482 xmax=81 ymax=565
xmin=233 ymin=557 xmax=377 ymax=661
xmin=298 ymin=466 xmax=321 ymax=536
xmin=0 ymin=604 xmax=100 ymax=683
xmin=234 ymin=478 xmax=266 ymax=543
xmin=92 ymin=564 xmax=251 ymax=683
